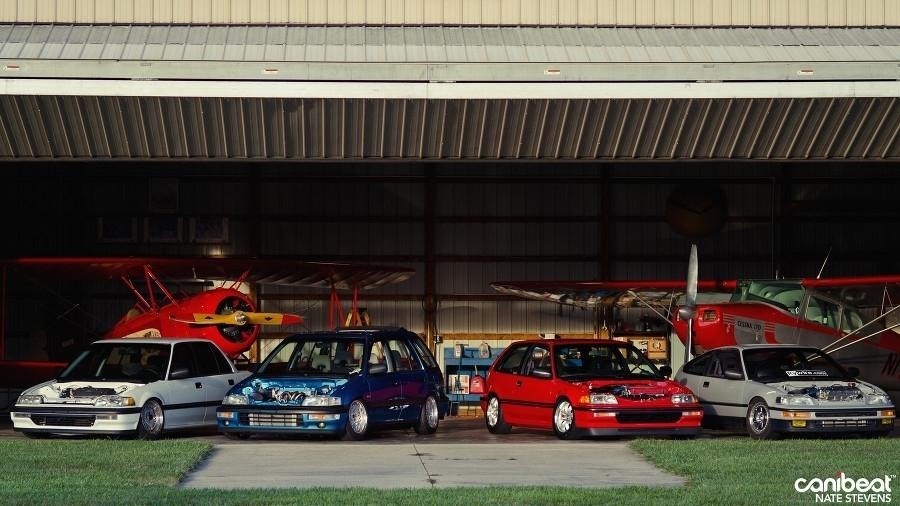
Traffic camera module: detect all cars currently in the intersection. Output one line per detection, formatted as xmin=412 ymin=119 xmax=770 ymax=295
xmin=11 ymin=337 xmax=254 ymax=441
xmin=216 ymin=326 xmax=450 ymax=441
xmin=480 ymin=339 xmax=704 ymax=440
xmin=676 ymin=342 xmax=897 ymax=439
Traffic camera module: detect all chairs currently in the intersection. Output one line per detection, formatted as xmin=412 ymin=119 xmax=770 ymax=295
xmin=311 ymin=350 xmax=402 ymax=373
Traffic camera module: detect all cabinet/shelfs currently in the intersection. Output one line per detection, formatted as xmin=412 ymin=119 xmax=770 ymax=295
xmin=444 ymin=348 xmax=507 ymax=416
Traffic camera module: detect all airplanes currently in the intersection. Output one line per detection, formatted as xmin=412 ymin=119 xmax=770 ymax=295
xmin=489 ymin=244 xmax=900 ymax=391
xmin=9 ymin=256 xmax=417 ymax=377
